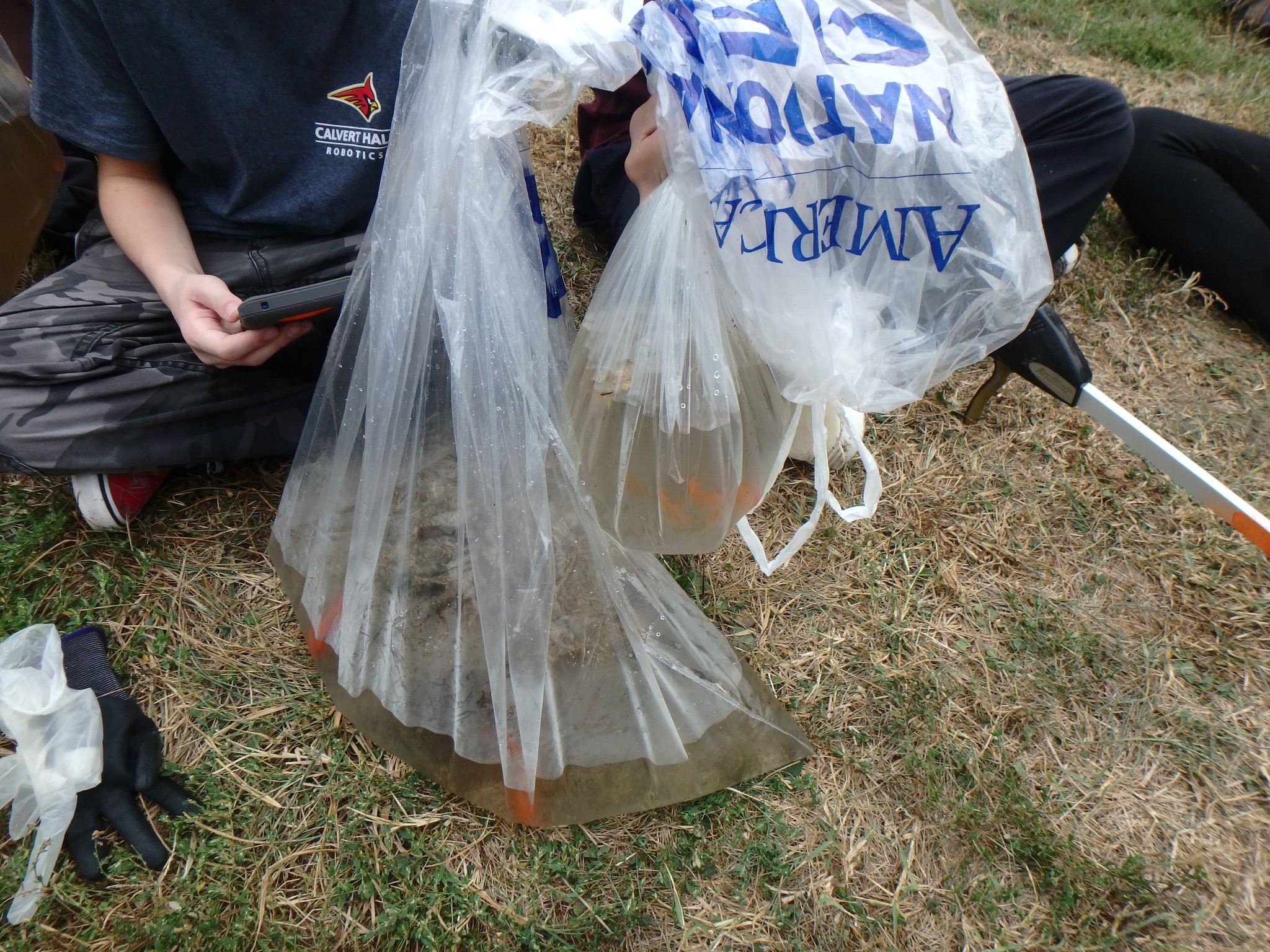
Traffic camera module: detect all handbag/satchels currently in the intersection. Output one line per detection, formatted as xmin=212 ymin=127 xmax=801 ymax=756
xmin=564 ymin=93 xmax=799 ymax=555
xmin=0 ymin=32 xmax=67 ymax=307
xmin=622 ymin=0 xmax=1055 ymax=579
xmin=266 ymin=1 xmax=818 ymax=829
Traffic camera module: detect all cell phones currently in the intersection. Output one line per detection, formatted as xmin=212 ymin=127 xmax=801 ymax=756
xmin=237 ymin=275 xmax=352 ymax=329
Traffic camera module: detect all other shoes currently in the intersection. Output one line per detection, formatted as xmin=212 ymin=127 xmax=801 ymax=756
xmin=786 ymin=400 xmax=865 ymax=475
xmin=71 ymin=466 xmax=173 ymax=533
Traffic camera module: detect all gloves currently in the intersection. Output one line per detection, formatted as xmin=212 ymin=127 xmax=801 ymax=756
xmin=60 ymin=624 xmax=209 ymax=882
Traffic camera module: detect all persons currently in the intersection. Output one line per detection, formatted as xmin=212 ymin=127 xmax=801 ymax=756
xmin=1116 ymin=106 xmax=1270 ymax=347
xmin=577 ymin=30 xmax=1134 ymax=471
xmin=0 ymin=1 xmax=449 ymax=537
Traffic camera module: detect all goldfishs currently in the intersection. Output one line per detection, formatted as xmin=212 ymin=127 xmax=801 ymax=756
xmin=501 ymin=731 xmax=542 ymax=824
xmin=663 ymin=470 xmax=762 ymax=523
xmin=307 ymin=587 xmax=345 ymax=661
